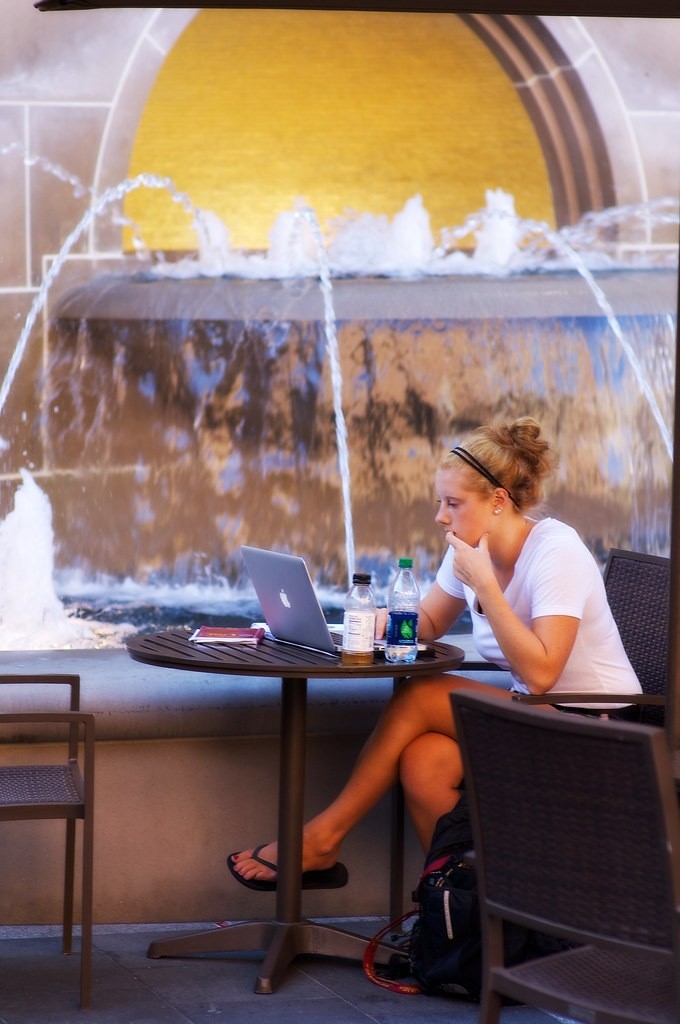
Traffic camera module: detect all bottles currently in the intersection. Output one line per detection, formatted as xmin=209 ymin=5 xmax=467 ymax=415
xmin=386 ymin=558 xmax=422 ymax=661
xmin=342 ymin=572 xmax=377 ymax=664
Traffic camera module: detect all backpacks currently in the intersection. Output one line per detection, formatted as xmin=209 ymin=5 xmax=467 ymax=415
xmin=363 ymin=792 xmax=563 ymax=1008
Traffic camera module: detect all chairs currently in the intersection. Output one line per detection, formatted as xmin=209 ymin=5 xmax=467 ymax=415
xmin=1 ymin=674 xmax=95 ymax=1010
xmin=446 ymin=548 xmax=671 ymax=731
xmin=446 ymin=689 xmax=679 ymax=1023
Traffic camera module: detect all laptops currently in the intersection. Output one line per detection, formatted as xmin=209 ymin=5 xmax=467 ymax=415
xmin=238 ymin=545 xmax=428 ymax=657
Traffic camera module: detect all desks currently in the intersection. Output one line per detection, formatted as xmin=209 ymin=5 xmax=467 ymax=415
xmin=125 ymin=625 xmax=466 ymax=993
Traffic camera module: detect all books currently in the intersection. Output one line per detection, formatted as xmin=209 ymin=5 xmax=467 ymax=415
xmin=188 ymin=626 xmax=264 ymax=645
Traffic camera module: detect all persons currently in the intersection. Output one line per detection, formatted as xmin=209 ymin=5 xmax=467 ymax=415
xmin=226 ymin=418 xmax=643 ymax=891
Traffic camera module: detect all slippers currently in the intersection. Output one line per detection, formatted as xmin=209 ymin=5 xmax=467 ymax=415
xmin=227 ymin=844 xmax=348 ymax=892
